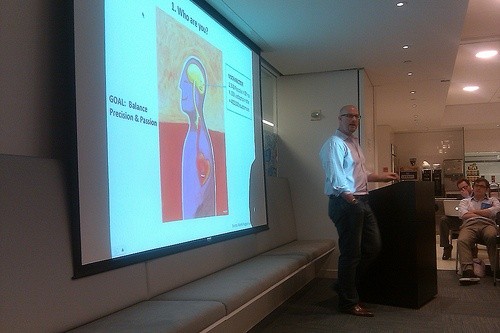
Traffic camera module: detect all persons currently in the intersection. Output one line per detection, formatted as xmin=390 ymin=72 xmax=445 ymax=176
xmin=439 ymin=178 xmax=474 ymax=260
xmin=322 ymin=106 xmax=400 ymax=316
xmin=458 ymin=178 xmax=500 ymax=283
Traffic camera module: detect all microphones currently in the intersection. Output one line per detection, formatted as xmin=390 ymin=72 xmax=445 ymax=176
xmin=391 ymin=154 xmax=400 ymax=181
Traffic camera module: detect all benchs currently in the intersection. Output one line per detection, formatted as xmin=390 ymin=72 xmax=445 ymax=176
xmin=64 ymin=237 xmax=336 ymax=333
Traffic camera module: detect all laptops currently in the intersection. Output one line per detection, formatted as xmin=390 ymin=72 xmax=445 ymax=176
xmin=443 ymin=200 xmax=462 ymax=217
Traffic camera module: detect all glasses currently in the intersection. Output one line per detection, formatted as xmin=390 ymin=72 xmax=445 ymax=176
xmin=475 ymin=184 xmax=486 ymax=188
xmin=341 ymin=113 xmax=359 ymax=119
xmin=459 ymin=186 xmax=469 ymax=191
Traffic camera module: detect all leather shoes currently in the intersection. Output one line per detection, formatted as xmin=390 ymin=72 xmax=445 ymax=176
xmin=350 ymin=303 xmax=374 ymax=317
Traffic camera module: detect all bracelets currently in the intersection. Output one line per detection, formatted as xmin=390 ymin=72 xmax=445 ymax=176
xmin=352 ymin=198 xmax=356 ymax=205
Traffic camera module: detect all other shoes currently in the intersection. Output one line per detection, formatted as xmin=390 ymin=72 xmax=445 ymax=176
xmin=494 ymin=272 xmax=500 ymax=282
xmin=459 ymin=269 xmax=481 ymax=283
xmin=472 ymin=258 xmax=482 ymax=264
xmin=442 ymin=245 xmax=454 ymax=260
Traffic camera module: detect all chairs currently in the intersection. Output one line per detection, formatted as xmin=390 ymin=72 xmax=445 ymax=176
xmin=455 ymin=207 xmax=497 ymax=286
